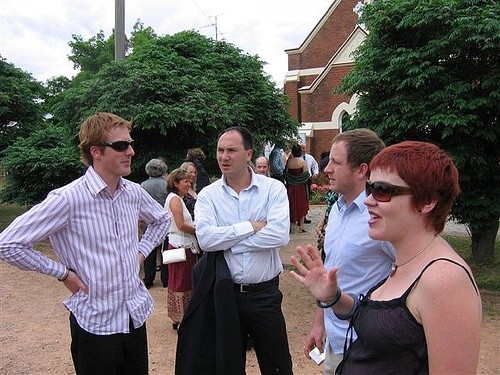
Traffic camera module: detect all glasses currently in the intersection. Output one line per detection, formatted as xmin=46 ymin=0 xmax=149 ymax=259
xmin=365 ymin=179 xmax=410 ymax=203
xmin=97 ymin=139 xmax=134 ymax=151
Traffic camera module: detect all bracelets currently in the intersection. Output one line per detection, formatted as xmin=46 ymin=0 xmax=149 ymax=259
xmin=334 ymin=293 xmax=358 ymax=320
xmin=57 ymin=266 xmax=69 ymax=282
xmin=316 ymin=287 xmax=341 ymax=308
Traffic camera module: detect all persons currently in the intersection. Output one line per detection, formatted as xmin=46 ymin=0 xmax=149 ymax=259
xmin=289 ymin=140 xmax=482 ymax=375
xmin=180 ymin=162 xmax=199 ymax=222
xmin=140 ymin=159 xmax=170 ymax=289
xmin=315 ymin=186 xmax=343 ymax=264
xmin=186 ymin=147 xmax=211 ymax=193
xmin=194 ymin=127 xmax=294 ymax=375
xmin=0 ymin=112 xmax=173 ymax=375
xmin=303 ymin=128 xmax=397 ymax=375
xmin=253 ymin=136 xmax=320 ymax=235
xmin=164 ymin=168 xmax=197 ymax=330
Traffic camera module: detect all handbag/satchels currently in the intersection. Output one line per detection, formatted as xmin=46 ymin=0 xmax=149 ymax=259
xmin=162 ymin=247 xmax=187 ymax=265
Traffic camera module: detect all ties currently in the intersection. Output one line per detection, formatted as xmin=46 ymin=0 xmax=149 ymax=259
xmin=303 ymin=156 xmax=306 ymax=160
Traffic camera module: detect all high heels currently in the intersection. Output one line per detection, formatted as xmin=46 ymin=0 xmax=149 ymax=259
xmin=290 ymin=226 xmax=294 ymax=234
xmin=299 ymin=227 xmax=308 ymax=233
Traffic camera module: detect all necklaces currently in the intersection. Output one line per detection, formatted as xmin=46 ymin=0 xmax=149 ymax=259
xmin=388 ymin=233 xmax=437 ymax=276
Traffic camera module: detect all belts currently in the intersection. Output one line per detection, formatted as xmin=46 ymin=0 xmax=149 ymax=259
xmin=235 ymin=276 xmax=278 ymax=292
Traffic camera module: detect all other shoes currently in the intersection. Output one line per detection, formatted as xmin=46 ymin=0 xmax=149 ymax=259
xmin=304 ymin=220 xmax=311 ymax=224
xmin=173 ymin=322 xmax=179 ymax=330
